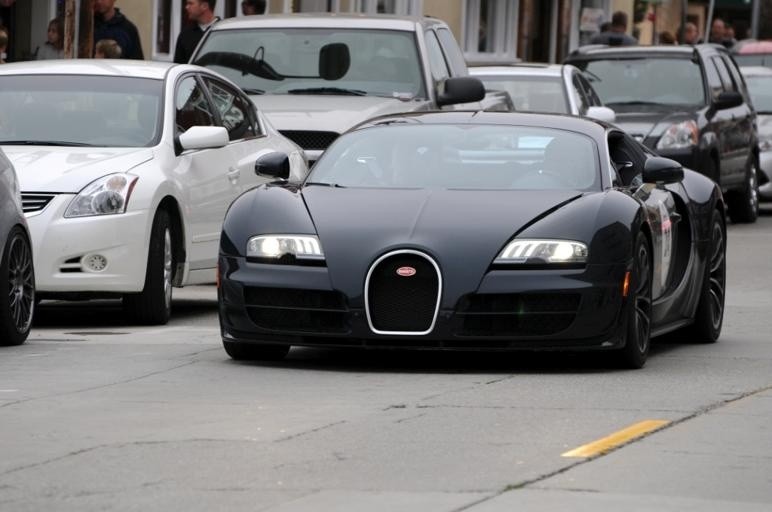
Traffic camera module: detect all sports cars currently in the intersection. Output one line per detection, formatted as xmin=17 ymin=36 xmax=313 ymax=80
xmin=215 ymin=109 xmax=725 ymax=371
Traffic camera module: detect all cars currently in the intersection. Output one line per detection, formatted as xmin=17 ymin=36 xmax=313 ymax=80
xmin=0 ymin=58 xmax=310 ymax=333
xmin=0 ymin=149 xmax=37 ymax=349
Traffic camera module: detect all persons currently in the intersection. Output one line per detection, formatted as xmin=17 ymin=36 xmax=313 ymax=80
xmin=241 ymin=1 xmax=266 ymax=16
xmin=174 ymin=0 xmax=221 ymax=64
xmin=678 ymin=22 xmax=699 ymax=45
xmin=659 ymin=31 xmax=675 ymax=44
xmin=94 ymin=1 xmax=144 ymax=60
xmin=1 ymin=29 xmax=10 ymax=65
xmin=710 ymin=19 xmax=735 ymax=48
xmin=28 ymin=15 xmax=66 ymax=60
xmin=726 ymin=26 xmax=739 ymax=45
xmin=94 ymin=38 xmax=122 ymax=59
xmin=599 ymin=21 xmax=612 ymax=31
xmin=588 ymin=11 xmax=640 ymax=47
